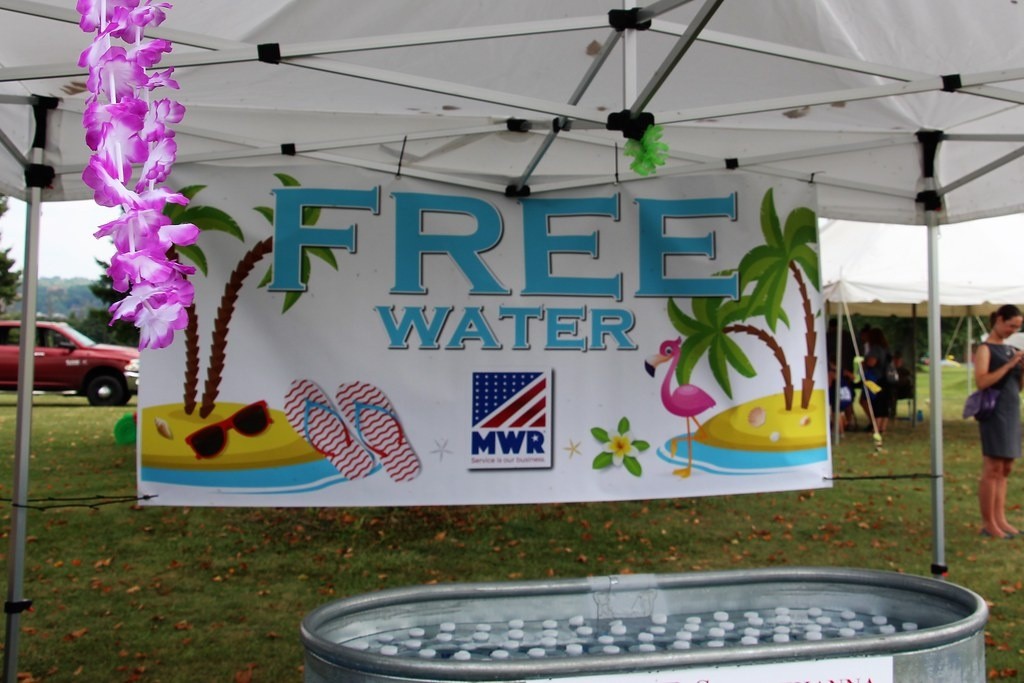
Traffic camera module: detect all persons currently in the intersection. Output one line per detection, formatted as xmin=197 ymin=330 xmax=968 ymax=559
xmin=973 ymin=304 xmax=1024 ymax=539
xmin=827 ymin=317 xmax=914 ymax=438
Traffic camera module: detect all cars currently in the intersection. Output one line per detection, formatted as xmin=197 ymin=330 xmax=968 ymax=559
xmin=0 ymin=321 xmax=140 ymax=408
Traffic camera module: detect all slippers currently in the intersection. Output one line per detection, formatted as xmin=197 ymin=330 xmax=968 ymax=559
xmin=335 ymin=380 xmax=421 ymax=484
xmin=284 ymin=378 xmax=376 ymax=482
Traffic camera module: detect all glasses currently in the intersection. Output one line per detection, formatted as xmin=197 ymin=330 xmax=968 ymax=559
xmin=185 ymin=400 xmax=274 ymax=459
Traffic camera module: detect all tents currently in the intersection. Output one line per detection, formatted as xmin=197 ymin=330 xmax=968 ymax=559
xmin=0 ymin=0 xmax=1024 ymax=683
xmin=820 ymin=213 xmax=1024 ymax=452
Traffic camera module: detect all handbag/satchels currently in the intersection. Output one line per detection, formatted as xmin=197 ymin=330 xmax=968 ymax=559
xmin=887 ymin=360 xmax=899 ymax=382
xmin=963 ymin=387 xmax=999 ymax=423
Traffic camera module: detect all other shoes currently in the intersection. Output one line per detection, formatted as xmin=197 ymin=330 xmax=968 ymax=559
xmin=1004 ymin=528 xmax=1024 ymax=537
xmin=980 ymin=529 xmax=1014 ymax=539
xmin=831 ymin=432 xmax=845 ymax=439
xmin=880 ymin=429 xmax=887 ymax=436
